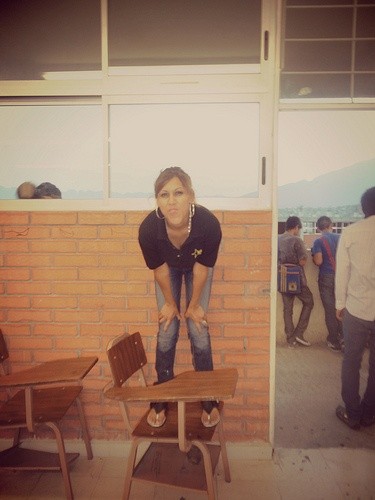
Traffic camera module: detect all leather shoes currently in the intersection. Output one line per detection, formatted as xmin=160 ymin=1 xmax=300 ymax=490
xmin=361 ymin=416 xmax=375 ymax=426
xmin=336 ymin=406 xmax=360 ymax=430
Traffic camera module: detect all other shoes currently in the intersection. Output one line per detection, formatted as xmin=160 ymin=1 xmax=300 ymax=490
xmin=295 ymin=332 xmax=310 ymax=345
xmin=338 ymin=337 xmax=345 ymax=347
xmin=328 ymin=342 xmax=341 ymax=352
xmin=288 ymin=340 xmax=296 ymax=348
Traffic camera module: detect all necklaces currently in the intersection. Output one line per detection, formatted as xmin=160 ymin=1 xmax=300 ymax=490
xmin=188 ymin=203 xmax=192 ymax=235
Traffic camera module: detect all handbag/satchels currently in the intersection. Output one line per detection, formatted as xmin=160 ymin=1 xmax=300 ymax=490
xmin=277 ymin=265 xmax=301 ymax=294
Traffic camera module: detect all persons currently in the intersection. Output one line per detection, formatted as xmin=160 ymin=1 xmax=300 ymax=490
xmin=32 ymin=182 xmax=63 ymax=198
xmin=311 ymin=215 xmax=345 ymax=352
xmin=333 ymin=187 xmax=375 ymax=427
xmin=17 ymin=182 xmax=35 ymax=198
xmin=137 ymin=168 xmax=221 ymax=428
xmin=278 ymin=216 xmax=314 ymax=348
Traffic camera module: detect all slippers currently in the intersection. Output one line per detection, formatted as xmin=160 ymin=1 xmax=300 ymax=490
xmin=147 ymin=404 xmax=167 ymax=428
xmin=201 ymin=403 xmax=220 ymax=428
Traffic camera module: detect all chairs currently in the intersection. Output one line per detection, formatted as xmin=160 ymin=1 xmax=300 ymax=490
xmin=103 ymin=331 xmax=239 ymax=500
xmin=0 ymin=329 xmax=99 ymax=500
